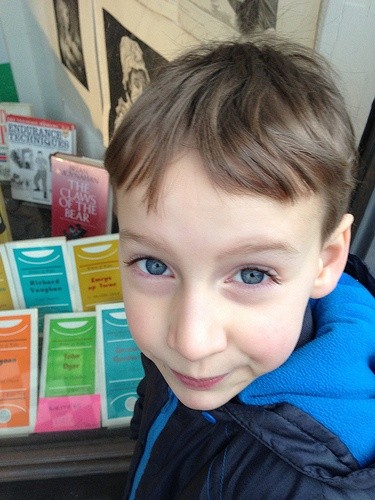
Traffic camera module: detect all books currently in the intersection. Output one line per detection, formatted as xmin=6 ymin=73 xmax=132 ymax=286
xmin=6 ymin=114 xmax=78 ymax=206
xmin=0 ymin=102 xmax=32 ymax=181
xmin=1 ymin=232 xmax=148 ymax=438
xmin=49 ymin=152 xmax=114 ymax=241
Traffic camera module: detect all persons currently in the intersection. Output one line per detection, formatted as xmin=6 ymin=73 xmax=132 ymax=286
xmin=103 ymin=37 xmax=375 ymax=500
